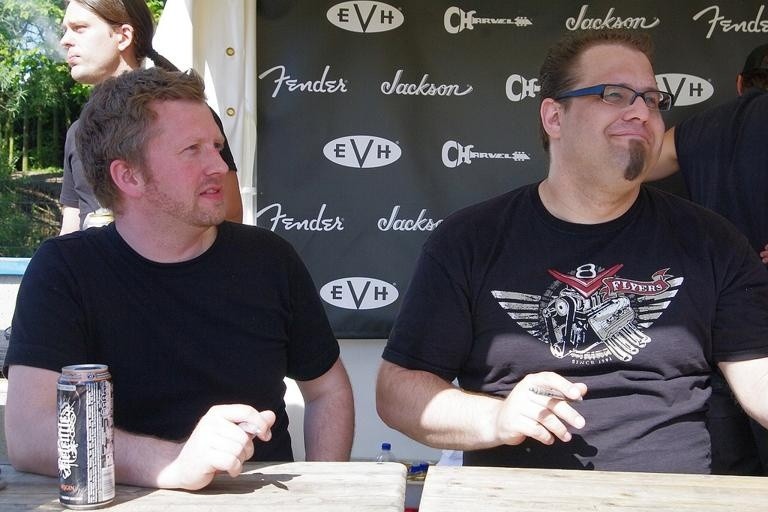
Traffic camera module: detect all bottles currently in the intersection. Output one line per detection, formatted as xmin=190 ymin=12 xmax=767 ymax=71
xmin=377 ymin=443 xmax=395 ymax=461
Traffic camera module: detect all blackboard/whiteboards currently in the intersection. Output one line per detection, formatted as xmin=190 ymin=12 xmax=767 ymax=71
xmin=259 ymin=0 xmax=767 ymax=359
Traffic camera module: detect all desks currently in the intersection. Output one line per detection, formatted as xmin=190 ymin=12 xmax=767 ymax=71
xmin=0 ymin=461 xmax=408 ymax=511
xmin=418 ymin=463 xmax=767 ymax=512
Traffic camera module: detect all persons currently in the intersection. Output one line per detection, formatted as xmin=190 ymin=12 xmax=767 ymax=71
xmin=3 ymin=68 xmax=355 ymax=493
xmin=375 ymin=30 xmax=768 ymax=476
xmin=639 ymin=44 xmax=767 ymax=476
xmin=57 ymin=0 xmax=244 ymax=235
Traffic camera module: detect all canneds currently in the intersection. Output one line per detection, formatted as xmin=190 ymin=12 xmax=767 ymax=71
xmin=56 ymin=365 xmax=116 ymax=511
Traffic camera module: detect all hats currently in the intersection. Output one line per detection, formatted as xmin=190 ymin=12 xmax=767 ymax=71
xmin=741 ymin=43 xmax=768 ymax=74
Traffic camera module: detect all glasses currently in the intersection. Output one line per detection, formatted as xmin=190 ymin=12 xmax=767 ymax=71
xmin=556 ymin=84 xmax=675 ymax=112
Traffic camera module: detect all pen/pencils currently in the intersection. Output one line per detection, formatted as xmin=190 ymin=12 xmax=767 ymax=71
xmin=528 ymin=387 xmax=583 ymax=401
xmin=409 ymin=463 xmax=429 ymax=473
xmin=237 ymin=421 xmax=265 ymax=435
